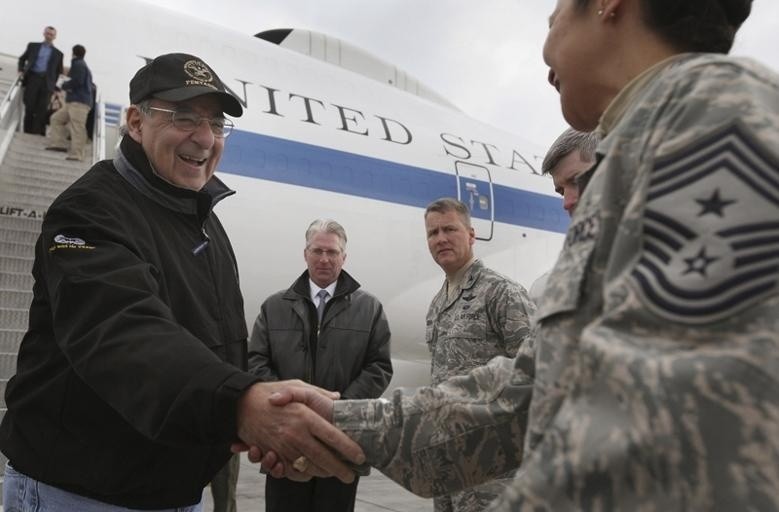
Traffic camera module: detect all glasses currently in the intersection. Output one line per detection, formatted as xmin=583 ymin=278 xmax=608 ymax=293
xmin=150 ymin=106 xmax=234 ymax=138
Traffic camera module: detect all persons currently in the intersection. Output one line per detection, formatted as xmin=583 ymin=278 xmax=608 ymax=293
xmin=17 ymin=26 xmax=65 ymax=136
xmin=0 ymin=52 xmax=367 ymax=512
xmin=42 ymin=45 xmax=94 ymax=161
xmin=543 ymin=125 xmax=601 ymax=222
xmin=246 ymin=216 xmax=394 ymax=512
xmin=229 ymin=0 xmax=779 ymax=511
xmin=423 ymin=197 xmax=539 ymax=511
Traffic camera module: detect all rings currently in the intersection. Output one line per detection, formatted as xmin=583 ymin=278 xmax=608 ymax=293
xmin=290 ymin=454 xmax=311 ymax=475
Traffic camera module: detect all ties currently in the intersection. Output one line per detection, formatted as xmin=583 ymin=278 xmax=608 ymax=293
xmin=315 ymin=290 xmax=328 ymax=330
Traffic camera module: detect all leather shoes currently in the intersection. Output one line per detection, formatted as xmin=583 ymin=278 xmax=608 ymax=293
xmin=46 ymin=146 xmax=67 ymax=152
xmin=65 ymin=157 xmax=78 ymax=160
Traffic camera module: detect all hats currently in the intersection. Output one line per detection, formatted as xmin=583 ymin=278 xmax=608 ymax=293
xmin=129 ymin=53 xmax=244 ymax=118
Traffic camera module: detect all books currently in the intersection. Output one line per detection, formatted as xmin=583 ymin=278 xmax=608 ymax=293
xmin=55 ymin=73 xmax=72 ymax=93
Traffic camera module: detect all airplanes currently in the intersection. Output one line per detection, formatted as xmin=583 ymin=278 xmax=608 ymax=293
xmin=2 ymin=2 xmax=582 ymax=405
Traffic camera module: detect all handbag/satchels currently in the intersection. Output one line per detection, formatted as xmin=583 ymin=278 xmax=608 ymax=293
xmin=46 ymin=96 xmax=62 ymax=125
